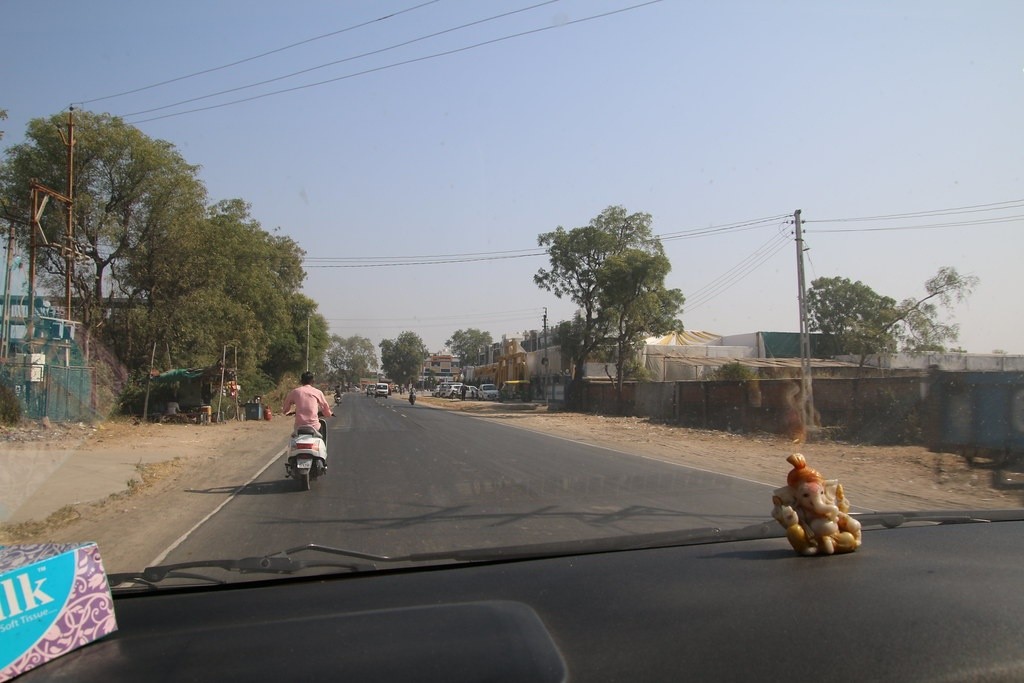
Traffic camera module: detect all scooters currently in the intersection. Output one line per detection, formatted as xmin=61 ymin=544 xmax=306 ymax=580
xmin=284 ymin=411 xmax=337 ymax=490
xmin=336 ymin=396 xmax=341 ymax=406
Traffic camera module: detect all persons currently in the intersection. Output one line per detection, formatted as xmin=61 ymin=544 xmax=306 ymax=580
xmin=408 ymin=385 xmax=416 ymax=398
xmin=460 ymin=383 xmax=467 ymax=401
xmin=334 ymin=385 xmax=342 ymax=401
xmin=168 ymin=399 xmax=182 ymax=417
xmin=282 ymin=371 xmax=333 ymax=435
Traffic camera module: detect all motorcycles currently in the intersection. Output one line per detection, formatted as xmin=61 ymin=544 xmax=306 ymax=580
xmin=497 ymin=381 xmax=533 ymax=402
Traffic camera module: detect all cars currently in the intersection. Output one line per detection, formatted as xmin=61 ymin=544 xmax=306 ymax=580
xmin=431 ymin=381 xmax=466 ymax=399
xmin=477 ymin=384 xmax=501 ymax=402
xmin=457 ymin=386 xmax=478 ymax=398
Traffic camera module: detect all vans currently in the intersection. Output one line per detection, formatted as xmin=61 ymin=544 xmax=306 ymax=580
xmin=374 ymin=383 xmax=389 ymax=399
xmin=366 ymin=384 xmax=375 ymax=397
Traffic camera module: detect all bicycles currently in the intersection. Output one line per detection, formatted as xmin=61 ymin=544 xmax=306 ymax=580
xmin=408 ymin=392 xmax=417 ymax=405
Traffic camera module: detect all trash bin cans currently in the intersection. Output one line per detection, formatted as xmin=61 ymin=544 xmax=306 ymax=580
xmin=245 ymin=403 xmax=265 ymax=420
